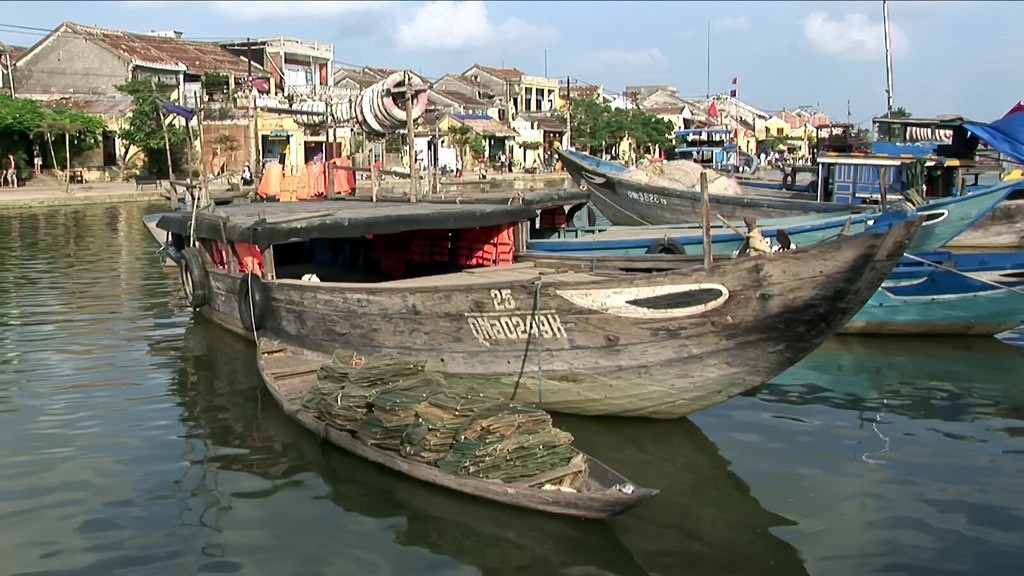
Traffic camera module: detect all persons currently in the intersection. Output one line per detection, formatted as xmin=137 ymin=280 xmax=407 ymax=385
xmin=240 ymin=166 xmax=255 ymax=186
xmin=499 ymin=153 xmax=514 ymax=175
xmin=34 ymin=144 xmax=42 ymax=175
xmin=7 ymin=153 xmax=17 ymax=188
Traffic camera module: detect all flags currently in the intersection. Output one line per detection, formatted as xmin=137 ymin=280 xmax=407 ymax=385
xmin=732 ymin=77 xmax=737 ymax=85
xmin=730 ymin=90 xmax=736 ymax=97
xmin=708 ymin=103 xmax=717 ymax=117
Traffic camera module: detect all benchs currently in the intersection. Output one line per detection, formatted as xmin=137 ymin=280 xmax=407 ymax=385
xmin=134 ymin=175 xmax=163 ymax=191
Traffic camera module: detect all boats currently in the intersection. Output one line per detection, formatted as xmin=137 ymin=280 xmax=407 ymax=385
xmin=297 ymin=1 xmax=1024 ymax=337
xmin=255 ymin=336 xmax=662 ymax=520
xmin=141 ymin=187 xmax=929 ymax=520
xmin=672 ymin=18 xmax=758 ymax=175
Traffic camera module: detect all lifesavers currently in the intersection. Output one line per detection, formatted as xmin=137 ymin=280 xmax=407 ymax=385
xmin=236 ymin=272 xmax=267 ymax=333
xmin=293 ymin=70 xmax=428 ymax=137
xmin=177 ymin=248 xmax=210 ymax=308
xmin=645 ymin=239 xmax=685 ymax=254
xmin=906 ymin=127 xmax=953 ymax=142
